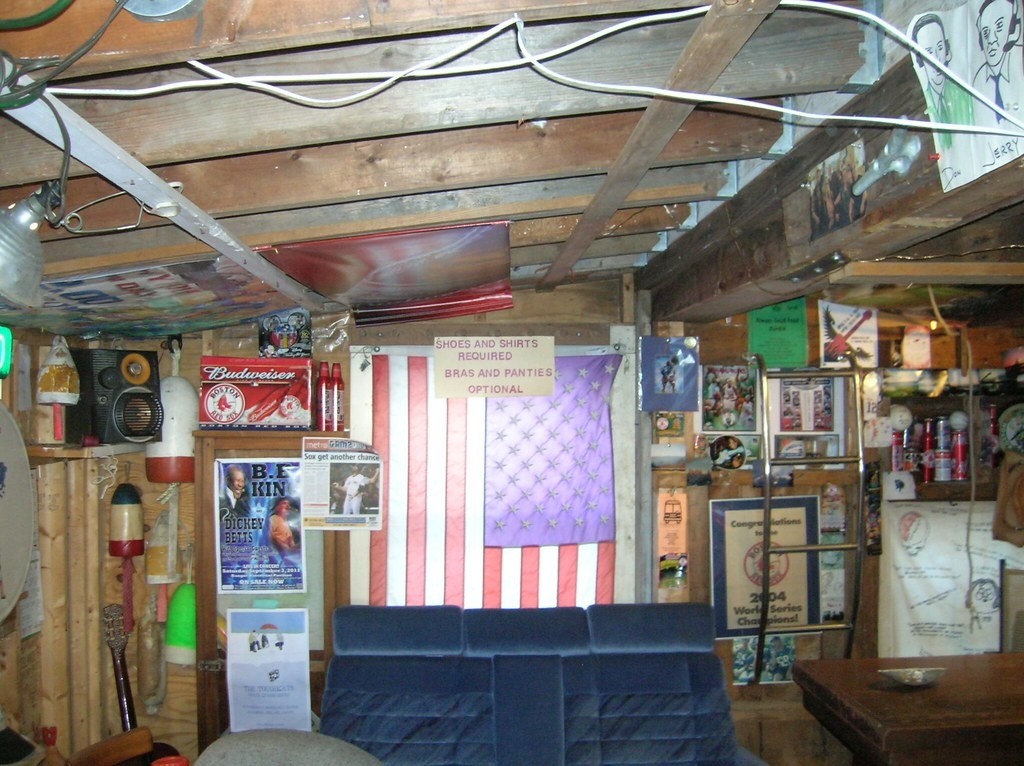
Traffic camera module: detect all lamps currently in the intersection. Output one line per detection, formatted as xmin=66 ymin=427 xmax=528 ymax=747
xmin=0 ymin=80 xmax=72 ymax=311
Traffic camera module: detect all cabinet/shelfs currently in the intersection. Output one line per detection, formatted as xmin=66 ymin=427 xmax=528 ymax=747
xmin=830 ymin=261 xmax=1024 ymax=501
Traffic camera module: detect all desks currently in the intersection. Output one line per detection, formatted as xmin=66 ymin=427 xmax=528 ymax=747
xmin=792 ymin=652 xmax=1024 ymax=766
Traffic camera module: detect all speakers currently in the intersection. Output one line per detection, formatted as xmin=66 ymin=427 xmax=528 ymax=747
xmin=66 ymin=348 xmax=164 ymax=445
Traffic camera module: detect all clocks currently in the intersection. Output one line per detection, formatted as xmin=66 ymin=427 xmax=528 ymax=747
xmin=999 ymin=404 xmax=1024 ymax=457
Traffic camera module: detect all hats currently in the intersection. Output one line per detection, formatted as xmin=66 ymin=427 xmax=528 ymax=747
xmin=770 ymin=636 xmax=781 ymax=643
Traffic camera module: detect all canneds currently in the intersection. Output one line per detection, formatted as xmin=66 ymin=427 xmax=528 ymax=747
xmin=921 ymin=452 xmax=934 ymax=482
xmin=903 ymin=450 xmax=920 ymax=481
xmin=935 ymin=416 xmax=950 ymax=448
xmin=922 ymin=419 xmax=935 ymax=451
xmin=935 ymin=449 xmax=952 ymax=481
xmin=951 ymin=430 xmax=970 ymax=480
xmin=903 ymin=421 xmax=922 ymax=449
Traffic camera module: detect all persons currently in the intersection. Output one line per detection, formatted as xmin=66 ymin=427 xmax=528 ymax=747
xmin=704 ymin=367 xmax=755 ymax=427
xmin=221 ymin=463 xmax=250 ymax=518
xmin=330 ymin=464 xmax=378 ymax=515
xmin=660 ymin=358 xmax=679 ymax=392
xmin=270 ymin=499 xmax=298 ymax=554
